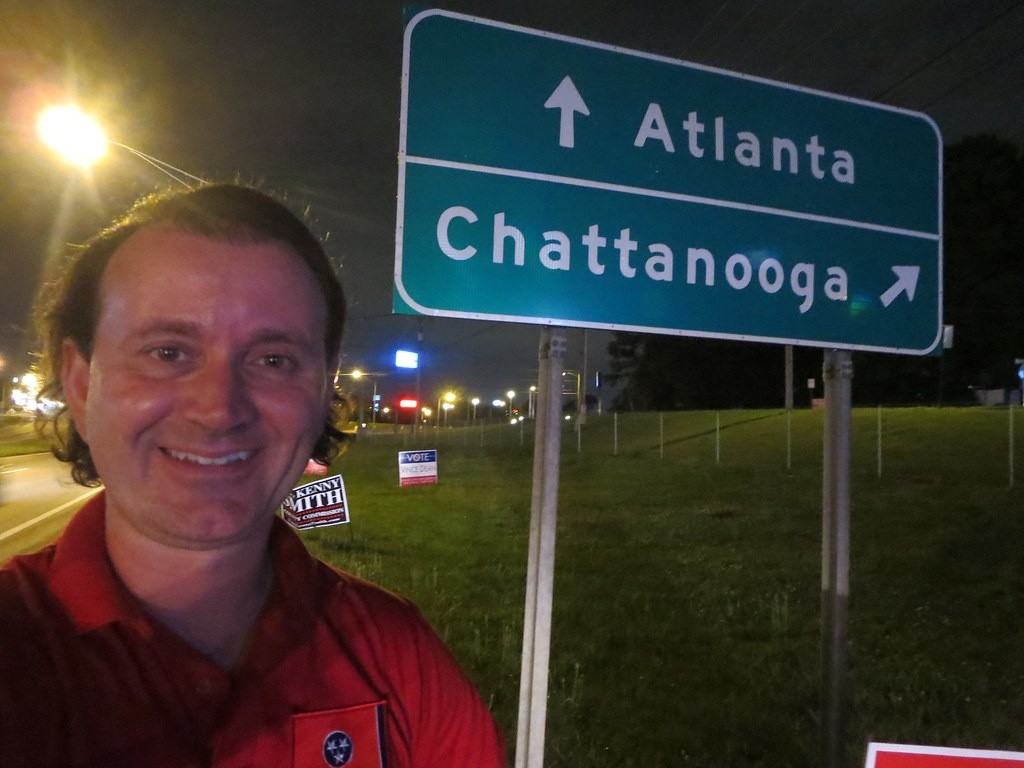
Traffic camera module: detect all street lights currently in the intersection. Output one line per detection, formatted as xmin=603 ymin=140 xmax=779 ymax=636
xmin=528 ymin=386 xmax=537 ymax=418
xmin=506 ymin=390 xmax=516 ymax=417
xmin=471 ymin=397 xmax=480 ymax=426
xmin=562 ymin=371 xmax=581 ymax=413
xmin=441 ymin=403 xmax=451 ymax=426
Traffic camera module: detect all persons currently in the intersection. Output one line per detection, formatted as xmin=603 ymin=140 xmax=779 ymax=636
xmin=0 ymin=183 xmax=508 ymax=768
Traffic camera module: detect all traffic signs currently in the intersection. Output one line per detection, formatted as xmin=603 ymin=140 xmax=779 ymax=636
xmin=392 ymin=5 xmax=946 ymax=359
